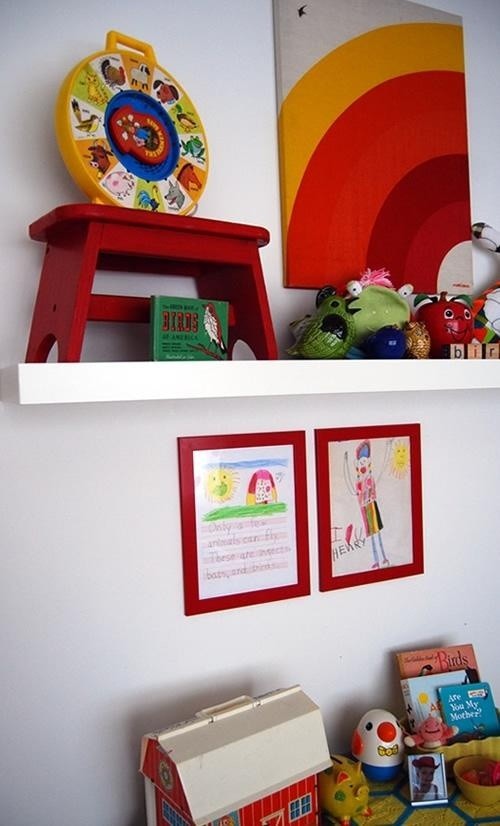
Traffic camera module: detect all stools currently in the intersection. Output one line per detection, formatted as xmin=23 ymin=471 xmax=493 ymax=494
xmin=22 ymin=200 xmax=283 ymax=364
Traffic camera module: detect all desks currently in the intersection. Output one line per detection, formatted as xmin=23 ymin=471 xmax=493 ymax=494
xmin=325 ymin=728 xmax=500 ymax=826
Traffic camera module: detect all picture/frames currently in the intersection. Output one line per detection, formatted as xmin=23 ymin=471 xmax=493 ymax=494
xmin=176 ymin=428 xmax=310 ymax=618
xmin=315 ymin=423 xmax=426 ymax=592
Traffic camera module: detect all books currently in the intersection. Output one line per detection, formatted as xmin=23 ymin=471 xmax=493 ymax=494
xmin=395 ymin=643 xmax=500 ymax=749
xmin=150 ymin=294 xmax=228 ymax=361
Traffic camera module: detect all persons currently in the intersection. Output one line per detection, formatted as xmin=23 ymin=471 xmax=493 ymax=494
xmin=413 ymin=757 xmax=441 ymax=802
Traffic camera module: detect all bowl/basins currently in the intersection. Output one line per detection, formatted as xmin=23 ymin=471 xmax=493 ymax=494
xmin=453 ymin=754 xmax=500 ymax=808
